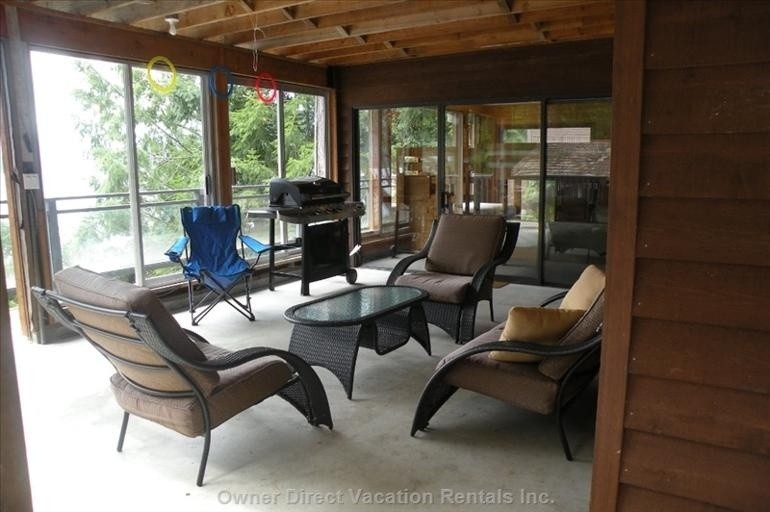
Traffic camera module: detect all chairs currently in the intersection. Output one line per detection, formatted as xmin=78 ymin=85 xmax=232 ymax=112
xmin=386 ymin=214 xmax=520 ymax=344
xmin=164 ymin=204 xmax=273 ymax=325
xmin=410 ymin=264 xmax=605 ymax=462
xmin=30 ymin=266 xmax=334 ymax=487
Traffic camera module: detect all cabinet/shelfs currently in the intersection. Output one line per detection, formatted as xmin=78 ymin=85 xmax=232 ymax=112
xmin=248 ymin=202 xmax=365 ymax=295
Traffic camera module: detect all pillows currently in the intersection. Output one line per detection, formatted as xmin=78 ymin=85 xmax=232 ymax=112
xmin=539 ymin=264 xmax=605 ymax=380
xmin=488 ymin=307 xmax=586 ymax=361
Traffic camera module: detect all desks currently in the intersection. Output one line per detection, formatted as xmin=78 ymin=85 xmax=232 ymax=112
xmin=283 ymin=285 xmax=431 ymax=400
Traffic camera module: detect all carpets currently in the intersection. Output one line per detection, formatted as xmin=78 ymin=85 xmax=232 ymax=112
xmin=493 ymin=282 xmax=509 ymax=288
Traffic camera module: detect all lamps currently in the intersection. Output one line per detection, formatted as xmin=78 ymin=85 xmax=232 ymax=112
xmin=164 ymin=15 xmax=180 ymax=35
